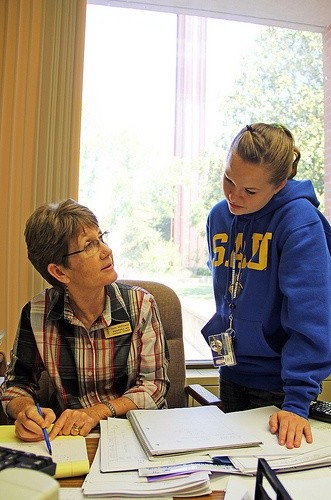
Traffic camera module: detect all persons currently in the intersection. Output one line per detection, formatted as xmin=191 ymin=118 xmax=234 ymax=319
xmin=200 ymin=123 xmax=331 ymax=448
xmin=0 ymin=198 xmax=170 ymax=442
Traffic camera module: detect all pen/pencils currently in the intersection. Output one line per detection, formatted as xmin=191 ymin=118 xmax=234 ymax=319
xmin=36 ymin=403 xmax=52 ymax=456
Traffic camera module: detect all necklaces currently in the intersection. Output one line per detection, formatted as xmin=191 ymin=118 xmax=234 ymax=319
xmin=229 ymin=247 xmax=245 ymax=318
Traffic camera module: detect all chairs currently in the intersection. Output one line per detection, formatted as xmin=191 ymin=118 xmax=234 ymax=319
xmin=115 ymin=280 xmax=221 ymax=409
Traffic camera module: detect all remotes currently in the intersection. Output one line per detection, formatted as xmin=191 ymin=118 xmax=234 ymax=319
xmin=308 ymin=400 xmax=331 ymax=423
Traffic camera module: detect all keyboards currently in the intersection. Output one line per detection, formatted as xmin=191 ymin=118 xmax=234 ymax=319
xmin=0 ymin=446 xmax=56 ymax=476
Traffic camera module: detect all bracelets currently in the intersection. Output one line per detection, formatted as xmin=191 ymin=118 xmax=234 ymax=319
xmin=100 ymin=401 xmax=116 ymax=418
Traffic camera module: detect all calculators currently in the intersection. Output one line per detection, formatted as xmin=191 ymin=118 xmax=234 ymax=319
xmin=308 ymin=399 xmax=331 ymax=424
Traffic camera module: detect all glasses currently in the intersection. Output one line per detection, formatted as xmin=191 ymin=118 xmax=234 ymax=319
xmin=67 ymin=229 xmax=110 ymax=257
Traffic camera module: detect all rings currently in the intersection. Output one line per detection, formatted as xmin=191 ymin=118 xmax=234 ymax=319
xmin=73 ymin=425 xmax=80 ymax=431
